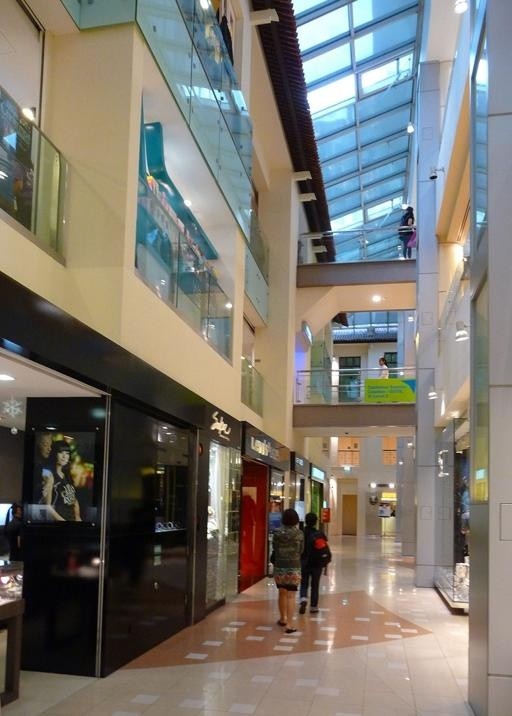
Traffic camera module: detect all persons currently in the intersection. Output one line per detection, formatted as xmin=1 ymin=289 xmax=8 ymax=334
xmin=42 ymin=440 xmax=84 ymax=522
xmin=299 ymin=512 xmax=332 ymax=613
xmin=34 ymin=431 xmax=52 ymax=506
xmin=398 ymin=206 xmax=415 ymax=258
xmin=272 ymin=508 xmax=305 ymax=633
xmin=4 ymin=504 xmax=23 ymax=560
xmin=377 ymin=357 xmax=388 ymax=379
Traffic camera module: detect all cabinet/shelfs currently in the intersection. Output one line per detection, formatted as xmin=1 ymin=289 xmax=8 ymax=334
xmin=1 ymin=561 xmax=27 ymax=706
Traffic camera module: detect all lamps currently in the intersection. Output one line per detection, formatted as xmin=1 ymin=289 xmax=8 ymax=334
xmin=428 ymin=166 xmax=445 ymax=181
xmin=454 ymin=319 xmax=470 ymax=344
xmin=406 ymin=122 xmax=417 ymax=134
xmin=427 ymin=385 xmax=444 ymax=401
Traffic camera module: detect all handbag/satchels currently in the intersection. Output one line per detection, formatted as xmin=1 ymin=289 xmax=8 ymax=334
xmin=312 ymin=532 xmax=331 ymax=565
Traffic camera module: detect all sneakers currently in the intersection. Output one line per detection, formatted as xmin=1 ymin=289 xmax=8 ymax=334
xmin=311 ymin=609 xmax=319 ymax=612
xmin=300 ymin=600 xmax=306 ymax=614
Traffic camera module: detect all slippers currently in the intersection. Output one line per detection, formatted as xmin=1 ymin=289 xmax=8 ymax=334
xmin=286 ymin=628 xmax=296 ymax=633
